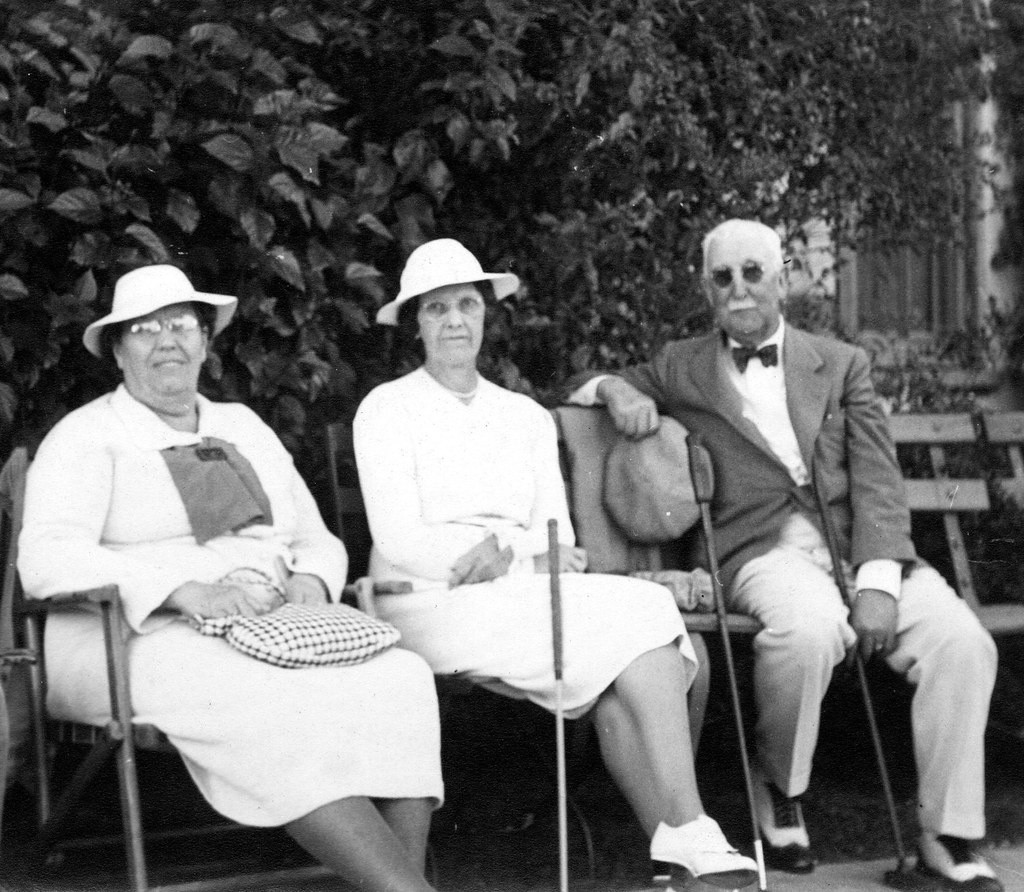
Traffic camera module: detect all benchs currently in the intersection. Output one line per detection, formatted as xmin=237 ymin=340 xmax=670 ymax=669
xmin=327 ymin=415 xmax=1024 ymax=883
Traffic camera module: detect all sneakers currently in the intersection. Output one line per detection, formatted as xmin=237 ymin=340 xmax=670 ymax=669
xmin=915 ymin=828 xmax=1006 ymax=892
xmin=747 ymin=757 xmax=814 ymax=872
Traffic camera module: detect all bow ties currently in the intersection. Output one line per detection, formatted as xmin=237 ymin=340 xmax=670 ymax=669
xmin=731 ymin=342 xmax=780 ymax=373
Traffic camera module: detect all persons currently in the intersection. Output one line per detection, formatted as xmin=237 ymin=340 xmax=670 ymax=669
xmin=353 ymin=239 xmax=759 ymax=892
xmin=558 ymin=217 xmax=1003 ymax=892
xmin=18 ymin=264 xmax=445 ymax=892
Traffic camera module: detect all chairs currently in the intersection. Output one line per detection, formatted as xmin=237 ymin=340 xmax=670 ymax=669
xmin=0 ymin=446 xmax=340 ymax=892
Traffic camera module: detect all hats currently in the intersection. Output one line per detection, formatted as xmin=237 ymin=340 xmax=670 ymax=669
xmin=603 ymin=417 xmax=700 ymax=543
xmin=376 ymin=238 xmax=521 ymax=323
xmin=83 ymin=265 xmax=239 ymax=357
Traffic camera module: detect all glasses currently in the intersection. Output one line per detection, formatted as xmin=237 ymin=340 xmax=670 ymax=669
xmin=420 ymin=295 xmax=484 ymax=320
xmin=708 ymin=261 xmax=784 ymax=286
xmin=120 ymin=311 xmax=206 ymax=339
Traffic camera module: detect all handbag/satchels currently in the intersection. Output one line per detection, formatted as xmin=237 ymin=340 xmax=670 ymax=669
xmin=187 ymin=569 xmax=399 ymax=669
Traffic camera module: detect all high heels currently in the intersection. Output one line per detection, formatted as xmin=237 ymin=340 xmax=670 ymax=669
xmin=647 ymin=813 xmax=759 ymax=888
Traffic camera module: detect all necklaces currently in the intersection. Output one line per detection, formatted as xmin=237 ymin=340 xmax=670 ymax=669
xmin=451 ymin=390 xmax=476 ymax=398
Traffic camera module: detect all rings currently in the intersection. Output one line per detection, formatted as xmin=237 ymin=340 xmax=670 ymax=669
xmin=876 ymin=645 xmax=882 ymax=649
